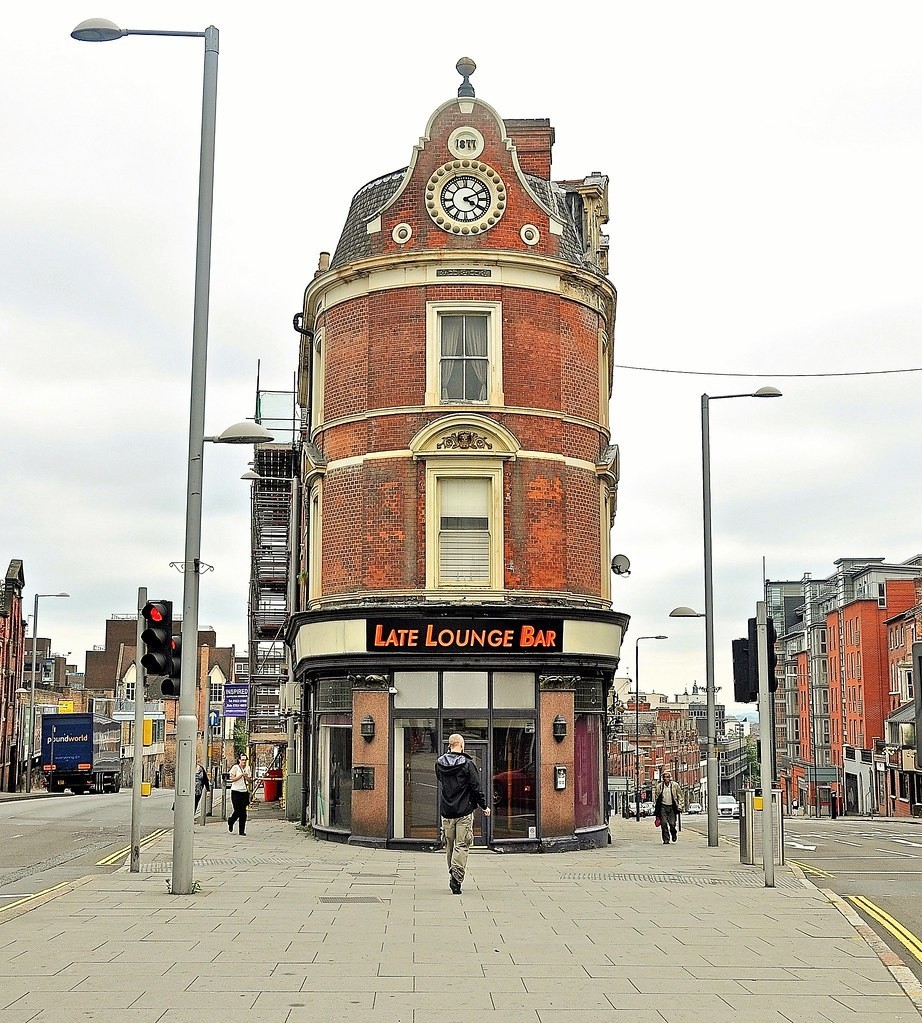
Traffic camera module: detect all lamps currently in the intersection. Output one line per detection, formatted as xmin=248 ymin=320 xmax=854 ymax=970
xmin=360 ymin=715 xmax=374 ymax=744
xmin=553 ymin=714 xmax=567 ymax=743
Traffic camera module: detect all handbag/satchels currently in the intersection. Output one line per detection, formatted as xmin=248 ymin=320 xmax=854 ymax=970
xmin=655 ymin=817 xmax=662 ymax=827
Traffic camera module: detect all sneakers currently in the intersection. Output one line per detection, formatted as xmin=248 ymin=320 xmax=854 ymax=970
xmin=450 ymin=877 xmax=461 ymax=894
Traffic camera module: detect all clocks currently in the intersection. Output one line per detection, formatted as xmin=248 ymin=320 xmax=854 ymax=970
xmin=441 ymin=176 xmax=491 ymax=223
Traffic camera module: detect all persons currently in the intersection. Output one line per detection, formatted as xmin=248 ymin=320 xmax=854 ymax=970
xmin=330 ymin=753 xmax=340 ymax=801
xmin=194 ymin=764 xmax=211 ymax=814
xmin=848 ymin=787 xmax=854 ymax=805
xmin=791 ymin=797 xmax=799 ymax=816
xmin=435 ymin=734 xmax=491 ymax=894
xmin=228 ymin=753 xmax=253 ymax=836
xmin=655 ymin=772 xmax=684 ymax=844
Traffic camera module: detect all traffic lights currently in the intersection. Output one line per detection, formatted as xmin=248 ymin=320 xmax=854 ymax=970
xmin=161 ymin=637 xmax=182 ymax=697
xmin=141 ymin=600 xmax=172 ymax=676
xmin=747 ymin=618 xmax=779 ymax=693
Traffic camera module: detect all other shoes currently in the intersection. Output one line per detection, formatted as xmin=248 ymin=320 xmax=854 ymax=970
xmin=663 ymin=840 xmax=669 ymax=844
xmin=228 ymin=817 xmax=233 ymax=832
xmin=672 ymin=834 xmax=676 ymax=842
xmin=239 ymin=832 xmax=246 ymax=835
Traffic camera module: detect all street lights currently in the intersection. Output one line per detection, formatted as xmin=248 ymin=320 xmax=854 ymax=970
xmin=669 ymin=386 xmax=783 ymax=847
xmin=635 ymin=635 xmax=668 ymax=821
xmin=13 ymin=592 xmax=70 ymax=793
xmin=70 ymin=16 xmax=273 ymax=890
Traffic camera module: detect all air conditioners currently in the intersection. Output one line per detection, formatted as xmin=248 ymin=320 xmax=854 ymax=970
xmin=279 ymin=682 xmax=301 ymax=714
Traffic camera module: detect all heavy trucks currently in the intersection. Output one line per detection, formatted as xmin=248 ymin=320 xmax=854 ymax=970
xmin=40 ymin=712 xmax=126 ymax=794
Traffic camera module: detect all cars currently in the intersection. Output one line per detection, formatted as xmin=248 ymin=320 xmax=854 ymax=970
xmin=716 ymin=795 xmax=739 ymax=820
xmin=492 ymin=760 xmax=536 ymax=810
xmin=222 ymin=767 xmax=267 ymax=787
xmin=628 ymin=801 xmax=656 ymax=819
xmin=688 ymin=803 xmax=702 ymax=815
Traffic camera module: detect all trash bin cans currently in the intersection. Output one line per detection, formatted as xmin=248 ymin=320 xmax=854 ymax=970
xmin=739 ymin=788 xmax=785 ymax=866
xmin=264 ymin=780 xmax=278 ymax=802
